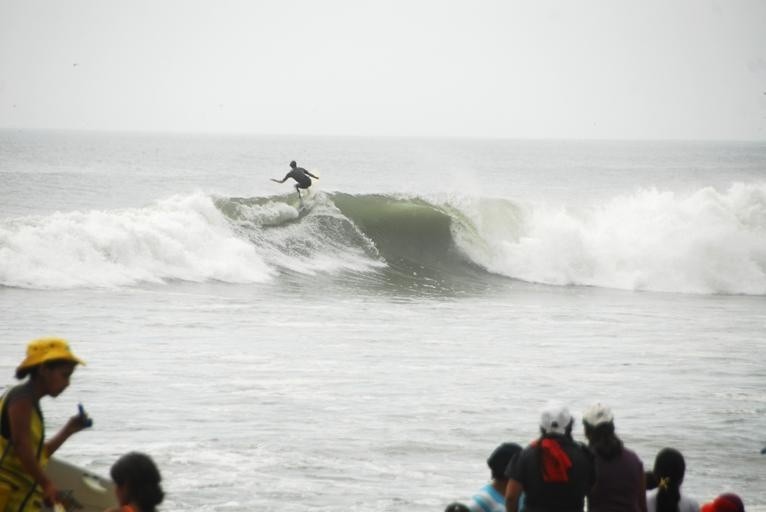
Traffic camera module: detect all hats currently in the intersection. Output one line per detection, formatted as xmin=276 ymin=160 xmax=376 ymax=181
xmin=538 ymin=399 xmax=573 ymax=435
xmin=582 ymin=400 xmax=614 ymax=427
xmin=700 ymin=494 xmax=746 ymax=512
xmin=15 ymin=338 xmax=87 ymax=380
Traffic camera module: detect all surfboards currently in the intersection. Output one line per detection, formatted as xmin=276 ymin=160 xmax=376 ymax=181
xmin=289 ymin=170 xmax=319 ymax=208
xmin=43 ymin=457 xmax=123 ymax=512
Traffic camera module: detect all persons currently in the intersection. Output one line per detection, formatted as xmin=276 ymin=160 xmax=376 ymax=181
xmin=268 ymin=160 xmax=319 ymax=198
xmin=0 ymin=335 xmax=95 ymax=512
xmin=643 ymin=445 xmax=702 ymax=512
xmin=576 ymin=401 xmax=649 ymax=511
xmin=700 ymin=491 xmax=746 ymax=512
xmin=102 ymin=450 xmax=166 ymax=512
xmin=499 ymin=400 xmax=596 ymax=511
xmin=459 ymin=440 xmax=528 ymax=512
